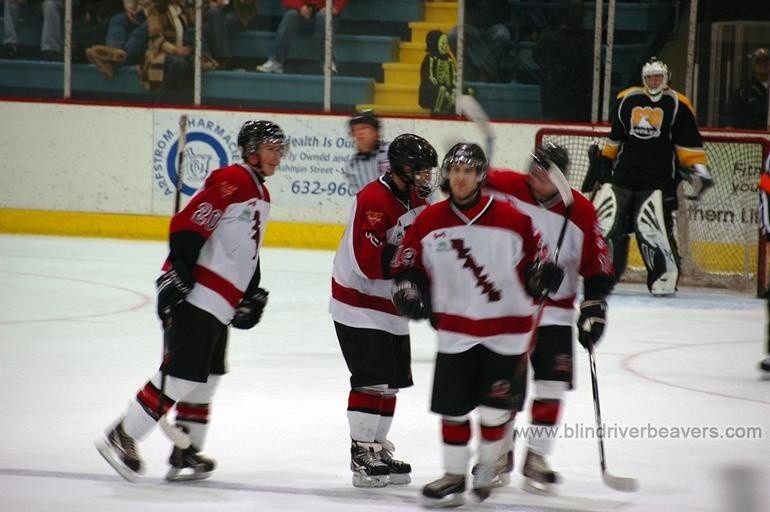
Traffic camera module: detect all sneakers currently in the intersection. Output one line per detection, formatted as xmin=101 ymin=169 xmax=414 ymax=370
xmin=93 ymin=45 xmax=127 ymax=63
xmin=86 ymin=49 xmax=115 ymax=77
xmin=349 ymin=444 xmax=389 ymax=475
xmin=423 ymin=474 xmax=465 ymax=499
xmin=257 ymin=59 xmax=283 ymax=74
xmin=170 ymin=445 xmax=215 ymax=472
xmin=472 ymin=462 xmax=497 ymax=491
xmin=472 ymin=449 xmax=512 ymax=474
xmin=523 ymin=451 xmax=556 ymax=482
xmin=321 ymin=58 xmax=338 ymax=77
xmin=375 ymin=440 xmax=411 ymax=473
xmin=109 ymin=423 xmax=141 ymax=471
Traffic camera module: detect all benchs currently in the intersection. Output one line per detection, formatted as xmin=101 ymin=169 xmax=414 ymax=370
xmin=0 ymin=0 xmax=680 ymax=120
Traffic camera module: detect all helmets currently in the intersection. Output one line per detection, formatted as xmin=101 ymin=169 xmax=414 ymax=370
xmin=388 ymin=134 xmax=441 ymax=199
xmin=236 ymin=120 xmax=290 ymax=157
xmin=440 ymin=142 xmax=487 ymax=193
xmin=350 ymin=108 xmax=379 ymax=129
xmin=642 ymin=58 xmax=671 ymax=100
xmin=534 ymin=143 xmax=568 ymax=177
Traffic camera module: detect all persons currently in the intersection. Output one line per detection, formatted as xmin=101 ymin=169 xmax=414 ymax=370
xmin=328 ymin=133 xmax=438 ymax=477
xmin=103 ymin=121 xmax=290 ymax=474
xmin=591 ymin=56 xmax=715 ymax=296
xmin=418 ymin=29 xmax=475 ymax=117
xmin=392 ymin=143 xmax=548 ymax=503
xmin=473 ymin=142 xmax=614 ymax=485
xmin=255 ymin=0 xmax=347 ymax=75
xmin=342 ymin=111 xmax=392 ymax=196
xmin=11 ymin=0 xmax=255 ymax=92
xmin=720 ymin=48 xmax=770 ymax=130
xmin=757 ymin=155 xmax=770 ymax=372
